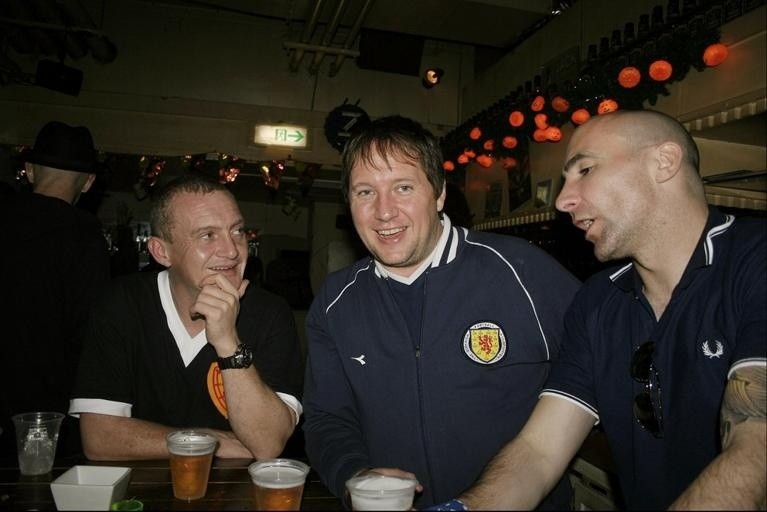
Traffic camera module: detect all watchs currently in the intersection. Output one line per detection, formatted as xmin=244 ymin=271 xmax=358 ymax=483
xmin=215 ymin=341 xmax=255 ymax=371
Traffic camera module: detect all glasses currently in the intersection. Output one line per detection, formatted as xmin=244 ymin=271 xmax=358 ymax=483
xmin=626 ymin=340 xmax=668 ymax=432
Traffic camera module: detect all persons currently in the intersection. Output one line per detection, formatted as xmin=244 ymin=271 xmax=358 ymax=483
xmin=1 ymin=118 xmax=124 ymax=435
xmin=404 ymin=107 xmax=767 ymax=511
xmin=298 ymin=112 xmax=585 ymax=510
xmin=65 ymin=160 xmax=310 ymax=463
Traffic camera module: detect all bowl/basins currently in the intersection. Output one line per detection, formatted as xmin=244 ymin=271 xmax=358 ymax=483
xmin=49 ymin=465 xmax=132 ymax=511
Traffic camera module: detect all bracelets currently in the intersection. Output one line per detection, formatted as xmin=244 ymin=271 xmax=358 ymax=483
xmin=412 ymin=499 xmax=471 ymax=510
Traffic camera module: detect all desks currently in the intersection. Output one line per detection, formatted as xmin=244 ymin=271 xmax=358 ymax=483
xmin=0 ymin=431 xmax=346 ymax=512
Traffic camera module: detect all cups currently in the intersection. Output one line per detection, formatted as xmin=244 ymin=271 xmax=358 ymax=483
xmin=345 ymin=474 xmax=419 ymax=512
xmin=13 ymin=410 xmax=66 ymax=476
xmin=247 ymin=457 xmax=311 ymax=511
xmin=166 ymin=430 xmax=219 ymax=500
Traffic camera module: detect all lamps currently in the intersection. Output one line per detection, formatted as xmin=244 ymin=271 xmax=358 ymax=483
xmin=421 ymin=68 xmax=444 ymax=89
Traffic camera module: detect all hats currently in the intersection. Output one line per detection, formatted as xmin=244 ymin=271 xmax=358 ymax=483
xmin=21 ymin=119 xmax=101 ymax=173
